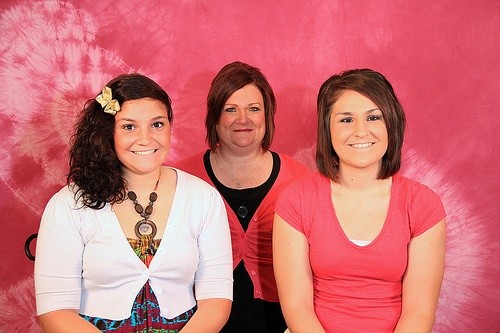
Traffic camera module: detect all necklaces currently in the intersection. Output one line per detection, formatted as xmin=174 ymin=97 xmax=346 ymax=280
xmin=121 ymin=165 xmax=166 ymax=242
xmin=214 ymin=148 xmax=265 ymax=190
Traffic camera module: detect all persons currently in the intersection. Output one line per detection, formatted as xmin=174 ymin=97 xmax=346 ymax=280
xmin=271 ymin=66 xmax=448 ymax=332
xmin=32 ymin=72 xmax=237 ymax=332
xmin=162 ymin=59 xmax=309 ymax=332
